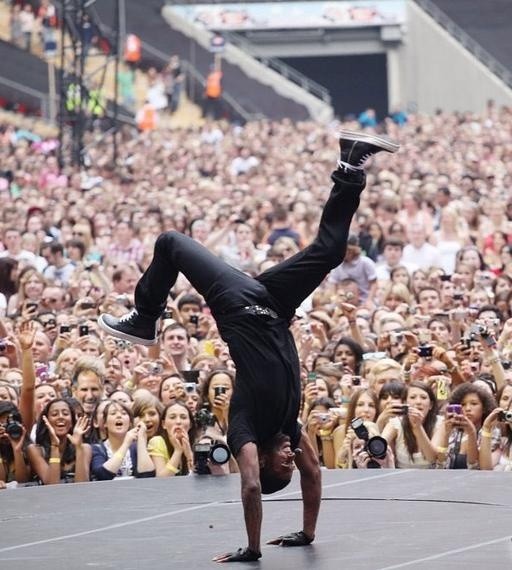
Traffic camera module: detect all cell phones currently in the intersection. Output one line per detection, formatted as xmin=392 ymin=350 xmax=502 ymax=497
xmin=448 ymin=404 xmax=462 ymax=427
xmin=392 ymin=404 xmax=408 ymax=416
xmin=191 ymin=316 xmax=198 ymax=328
xmin=214 ymin=387 xmax=225 ymax=405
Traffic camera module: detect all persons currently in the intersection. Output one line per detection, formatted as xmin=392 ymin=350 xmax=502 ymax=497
xmin=0 ymin=0 xmax=189 ymax=99
xmin=1 ymin=97 xmax=511 ymax=492
xmin=98 ymin=126 xmax=402 ymax=564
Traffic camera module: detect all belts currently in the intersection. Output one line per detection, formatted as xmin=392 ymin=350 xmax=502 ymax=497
xmin=223 ymin=305 xmax=279 ymax=319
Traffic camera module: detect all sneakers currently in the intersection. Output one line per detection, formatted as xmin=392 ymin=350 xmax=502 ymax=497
xmin=96 ymin=310 xmax=162 ymax=349
xmin=337 ymin=128 xmax=402 ymax=174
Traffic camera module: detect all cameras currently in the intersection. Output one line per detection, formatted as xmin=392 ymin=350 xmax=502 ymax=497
xmin=148 ymin=363 xmax=163 ymax=374
xmin=193 ymin=441 xmax=231 ymax=474
xmin=235 ymin=218 xmax=245 ymax=224
xmin=195 ymin=408 xmax=216 ymax=428
xmin=84 ymin=264 xmax=93 ymax=270
xmin=320 ymin=414 xmax=330 ymax=423
xmin=179 ymin=370 xmax=201 ymax=384
xmin=497 ymin=410 xmax=512 ymax=421
xmin=1 ymin=413 xmax=22 ymax=440
xmin=183 ymin=382 xmax=196 ymax=394
xmin=300 ymin=275 xmax=512 ymax=392
xmin=162 ymin=312 xmax=172 ymax=318
xmin=0 ymin=295 xmax=134 ymax=381
xmin=351 ymin=416 xmax=387 ymax=468
